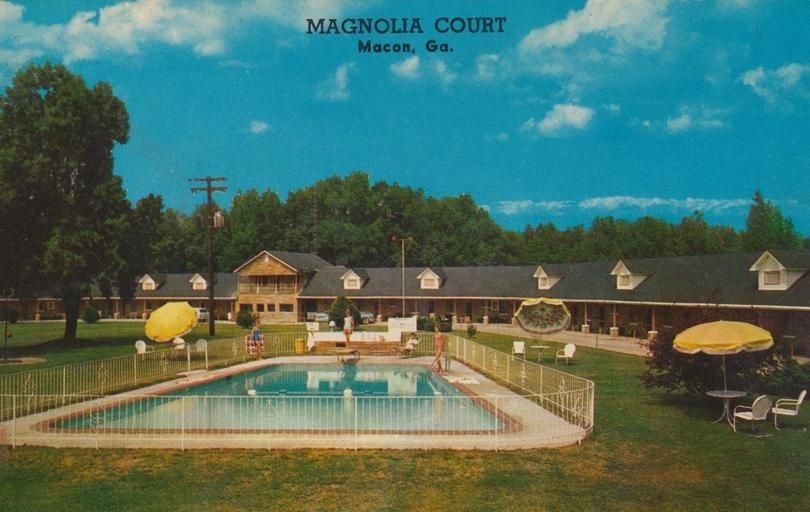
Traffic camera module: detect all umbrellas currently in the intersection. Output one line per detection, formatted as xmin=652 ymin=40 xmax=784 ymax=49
xmin=144 ymin=299 xmax=199 ymax=343
xmin=513 ymin=296 xmax=572 ymax=345
xmin=671 ymin=318 xmax=775 ymax=392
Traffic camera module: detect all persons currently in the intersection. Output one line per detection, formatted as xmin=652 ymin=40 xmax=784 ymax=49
xmin=251 ymin=319 xmax=264 ymax=360
xmin=343 ymin=308 xmax=355 ymax=351
xmin=434 ymin=326 xmax=445 ymax=374
xmin=399 ymin=331 xmax=418 ymax=353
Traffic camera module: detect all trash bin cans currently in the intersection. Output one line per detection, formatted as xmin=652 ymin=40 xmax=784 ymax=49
xmin=295 ymin=339 xmax=304 ymax=354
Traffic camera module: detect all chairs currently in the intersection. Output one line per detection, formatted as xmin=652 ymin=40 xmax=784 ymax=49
xmin=555 ymin=343 xmax=577 ymax=365
xmin=174 ymin=338 xmax=185 ymax=359
xmin=398 ymin=337 xmax=421 ymax=358
xmin=135 ymin=340 xmax=155 ymax=361
xmin=772 ymin=389 xmax=807 ymax=434
xmin=511 ymin=340 xmax=526 ymax=360
xmin=732 ymin=395 xmax=773 ymax=439
xmin=191 ymin=339 xmax=207 ymax=360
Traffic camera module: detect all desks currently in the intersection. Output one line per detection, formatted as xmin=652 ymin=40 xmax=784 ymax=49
xmin=706 ymin=389 xmax=745 ymax=432
xmin=530 ymin=345 xmax=550 ymax=364
